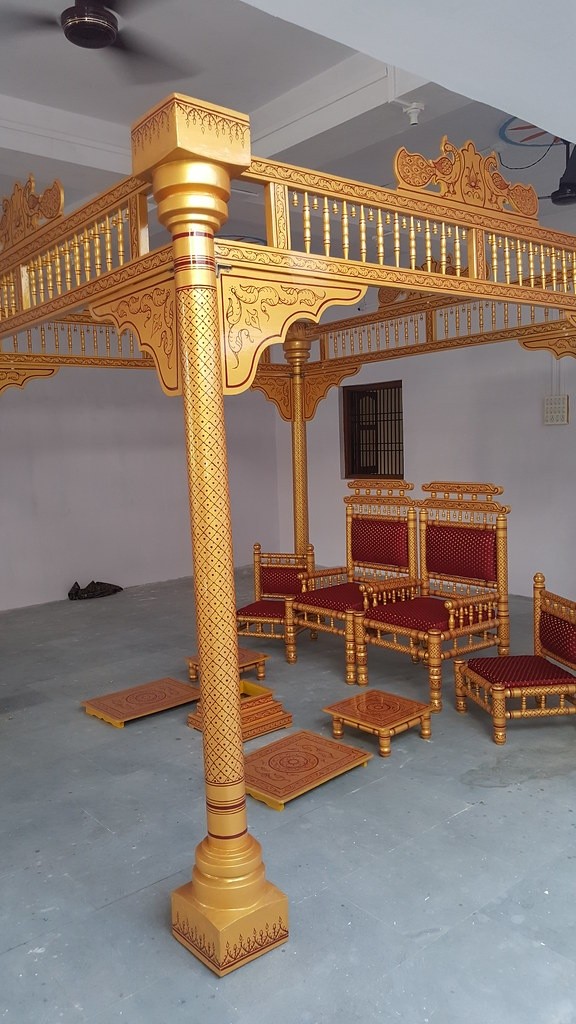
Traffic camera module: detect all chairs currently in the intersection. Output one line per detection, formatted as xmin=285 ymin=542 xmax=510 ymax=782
xmin=353 ymin=482 xmax=512 ymax=708
xmin=284 ymin=480 xmax=416 ymax=685
xmin=234 ymin=541 xmax=318 ymax=647
xmin=454 ymin=572 xmax=576 ymax=746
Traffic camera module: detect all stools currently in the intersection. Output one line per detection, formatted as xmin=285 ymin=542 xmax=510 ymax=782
xmin=321 ymin=687 xmax=435 ymax=759
xmin=183 ymin=647 xmax=271 ymax=682
